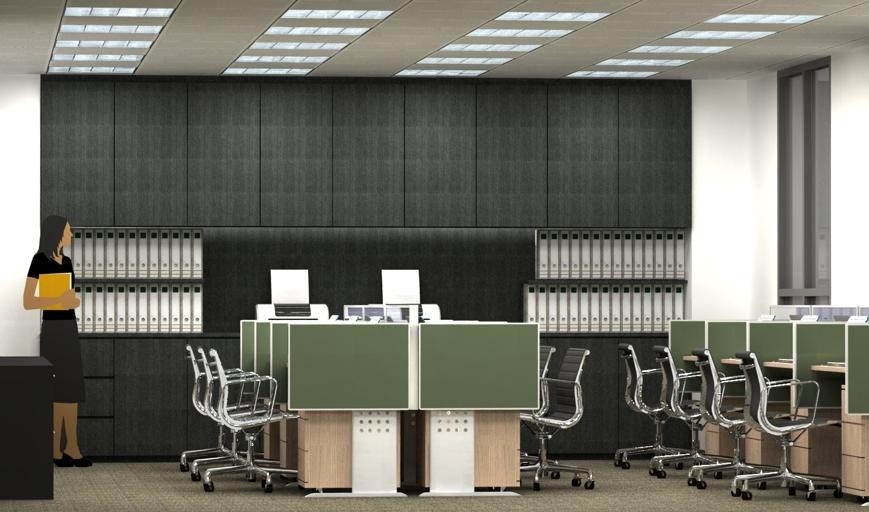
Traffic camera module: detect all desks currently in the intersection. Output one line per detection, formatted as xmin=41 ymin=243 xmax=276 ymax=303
xmin=239 ymin=317 xmax=540 ymax=497
xmin=668 ymin=320 xmax=867 ymax=496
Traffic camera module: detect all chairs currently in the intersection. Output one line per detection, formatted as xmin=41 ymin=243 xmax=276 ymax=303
xmin=732 ymin=351 xmax=844 ymax=502
xmin=617 ymin=342 xmax=686 ymax=479
xmin=650 ymin=343 xmax=723 ymax=482
xmin=521 ymin=348 xmax=595 ymax=491
xmin=687 ymin=349 xmax=770 ymax=490
xmin=178 ymin=346 xmax=301 ymax=495
xmin=521 ymin=345 xmax=561 ymax=480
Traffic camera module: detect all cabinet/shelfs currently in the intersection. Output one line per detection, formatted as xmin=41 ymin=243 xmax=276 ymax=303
xmin=617 ymin=77 xmax=692 ymax=229
xmin=520 ymin=331 xmax=619 ymax=458
xmin=75 ymin=332 xmax=241 ymax=463
xmin=547 ymin=77 xmax=616 ymax=230
xmin=40 ymin=74 xmax=114 ymax=231
xmin=404 ymin=79 xmax=476 ymax=229
xmin=0 ymin=355 xmax=55 ymax=500
xmin=475 ymin=78 xmax=547 ymax=230
xmin=260 ymin=77 xmax=335 ymax=228
xmin=187 ymin=80 xmax=258 ymax=231
xmin=114 ymin=78 xmax=188 ymax=231
xmin=616 ymin=328 xmax=694 ymax=455
xmin=333 ymin=80 xmax=406 ymax=227
